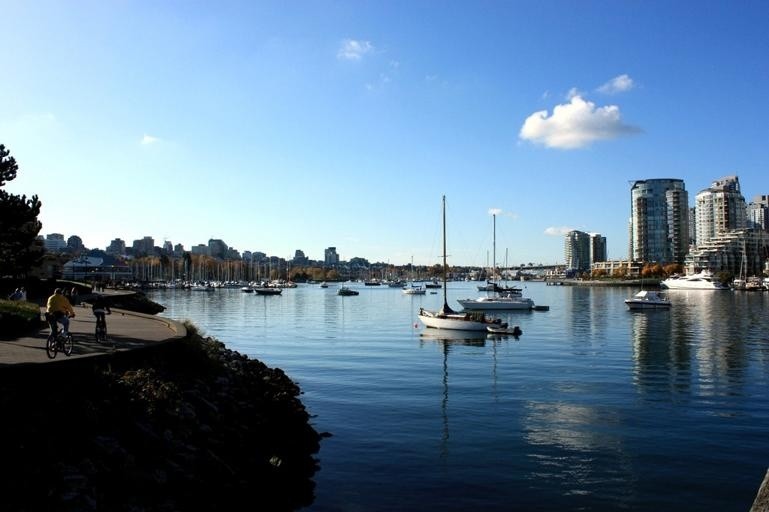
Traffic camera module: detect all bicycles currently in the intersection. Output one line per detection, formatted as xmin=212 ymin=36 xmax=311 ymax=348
xmin=95 ymin=312 xmax=110 ymax=344
xmin=46 ymin=316 xmax=73 ymax=359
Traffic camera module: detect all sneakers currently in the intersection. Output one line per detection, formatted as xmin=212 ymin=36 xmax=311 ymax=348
xmin=61 ymin=335 xmax=67 ymax=339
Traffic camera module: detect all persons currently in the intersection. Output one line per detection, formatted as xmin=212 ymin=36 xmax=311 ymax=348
xmin=91 ymin=294 xmax=111 ymax=330
xmin=91 ymin=279 xmax=106 ymax=292
xmin=70 ymin=284 xmax=78 ymax=306
xmin=61 ymin=287 xmax=67 ymax=296
xmin=19 ymin=287 xmax=27 ymax=301
xmin=9 ymin=287 xmax=22 ymax=301
xmin=45 ymin=288 xmax=75 ymax=350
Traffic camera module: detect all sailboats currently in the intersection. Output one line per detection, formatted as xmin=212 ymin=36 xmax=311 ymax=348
xmin=133 ymin=255 xmax=297 ymax=294
xmin=416 ymin=195 xmax=534 ymax=333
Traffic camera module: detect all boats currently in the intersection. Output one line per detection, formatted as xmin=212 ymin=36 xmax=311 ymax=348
xmin=728 ymin=239 xmax=769 ymax=291
xmin=337 ymin=284 xmax=359 ymax=296
xmin=625 ymin=291 xmax=671 ymax=310
xmin=320 ymin=282 xmax=328 ymax=287
xmin=661 ymin=269 xmax=730 ymax=290
xmin=364 ymin=278 xmax=426 ymax=295
